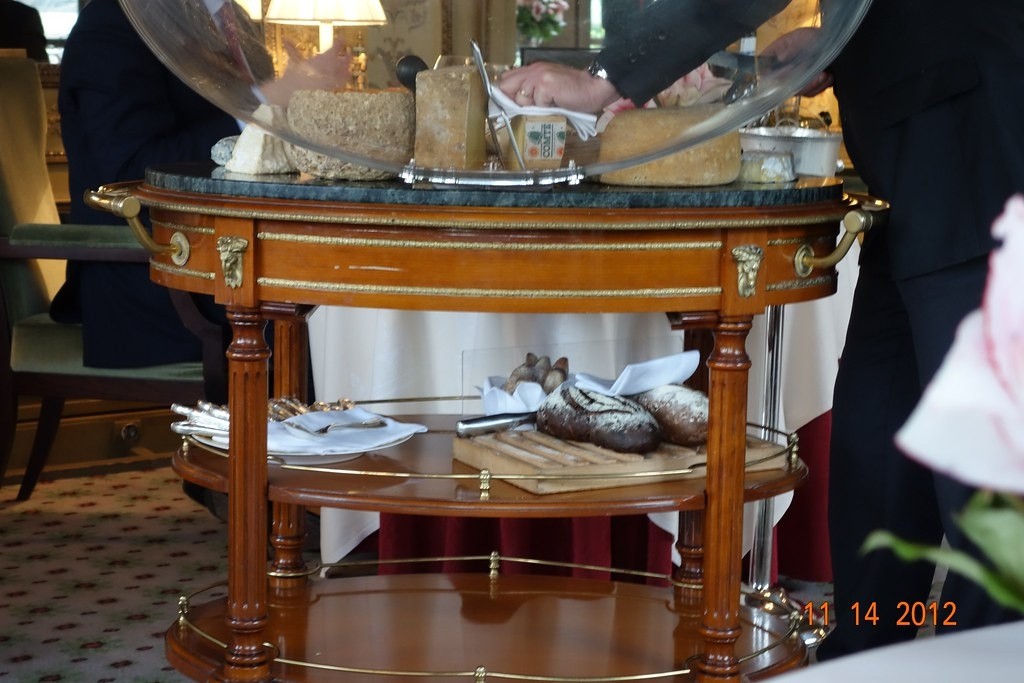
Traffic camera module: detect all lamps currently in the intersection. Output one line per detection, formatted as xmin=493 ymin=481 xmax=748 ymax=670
xmin=265 ymin=0 xmax=388 ymax=54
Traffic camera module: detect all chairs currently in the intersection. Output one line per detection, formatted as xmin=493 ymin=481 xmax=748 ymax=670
xmin=0 ymin=55 xmax=222 ymax=506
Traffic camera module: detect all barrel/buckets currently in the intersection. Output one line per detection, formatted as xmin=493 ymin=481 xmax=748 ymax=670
xmin=739 ymin=104 xmax=843 ymax=179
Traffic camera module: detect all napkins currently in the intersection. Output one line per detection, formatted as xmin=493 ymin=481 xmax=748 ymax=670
xmin=473 ymin=375 xmax=547 ymax=434
xmin=575 ymin=347 xmax=700 ymax=397
xmin=267 ymin=406 xmax=429 ymax=454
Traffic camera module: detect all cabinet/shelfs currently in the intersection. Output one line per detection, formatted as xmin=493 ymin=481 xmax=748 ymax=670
xmin=84 ymin=165 xmax=883 ymax=683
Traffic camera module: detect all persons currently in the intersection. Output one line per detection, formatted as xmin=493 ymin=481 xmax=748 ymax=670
xmin=57 ymin=1 xmax=323 ymax=550
xmin=0 ymin=0 xmax=49 ymax=63
xmin=494 ymin=0 xmax=1024 ymax=664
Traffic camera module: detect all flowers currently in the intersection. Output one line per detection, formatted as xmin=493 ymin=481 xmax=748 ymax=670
xmin=864 ymin=191 xmax=1024 ymax=612
xmin=516 ymin=0 xmax=569 ymax=47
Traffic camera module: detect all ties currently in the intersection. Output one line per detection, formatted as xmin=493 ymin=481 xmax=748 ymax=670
xmin=216 ymin=1 xmax=258 ymax=89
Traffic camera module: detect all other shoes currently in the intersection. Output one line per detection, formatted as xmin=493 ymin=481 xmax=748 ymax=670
xmin=301 ymin=511 xmax=321 ymax=550
xmin=182 ymin=480 xmax=228 ymax=523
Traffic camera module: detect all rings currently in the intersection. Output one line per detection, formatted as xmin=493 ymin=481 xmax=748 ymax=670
xmin=519 ymin=89 xmax=533 ymax=97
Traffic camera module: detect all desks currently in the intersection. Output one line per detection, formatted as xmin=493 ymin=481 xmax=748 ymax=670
xmin=307 ymin=222 xmax=862 ymax=582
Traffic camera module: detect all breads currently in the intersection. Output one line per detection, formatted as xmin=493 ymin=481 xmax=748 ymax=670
xmin=211 ymin=63 xmax=795 ymax=186
xmin=536 ymin=379 xmax=711 ymax=453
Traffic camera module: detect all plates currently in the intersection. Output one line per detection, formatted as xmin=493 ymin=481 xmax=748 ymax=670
xmin=192 ymin=433 xmax=415 ymax=465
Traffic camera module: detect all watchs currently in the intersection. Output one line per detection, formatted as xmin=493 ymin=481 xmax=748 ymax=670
xmin=588 ymin=62 xmax=609 ymax=79
